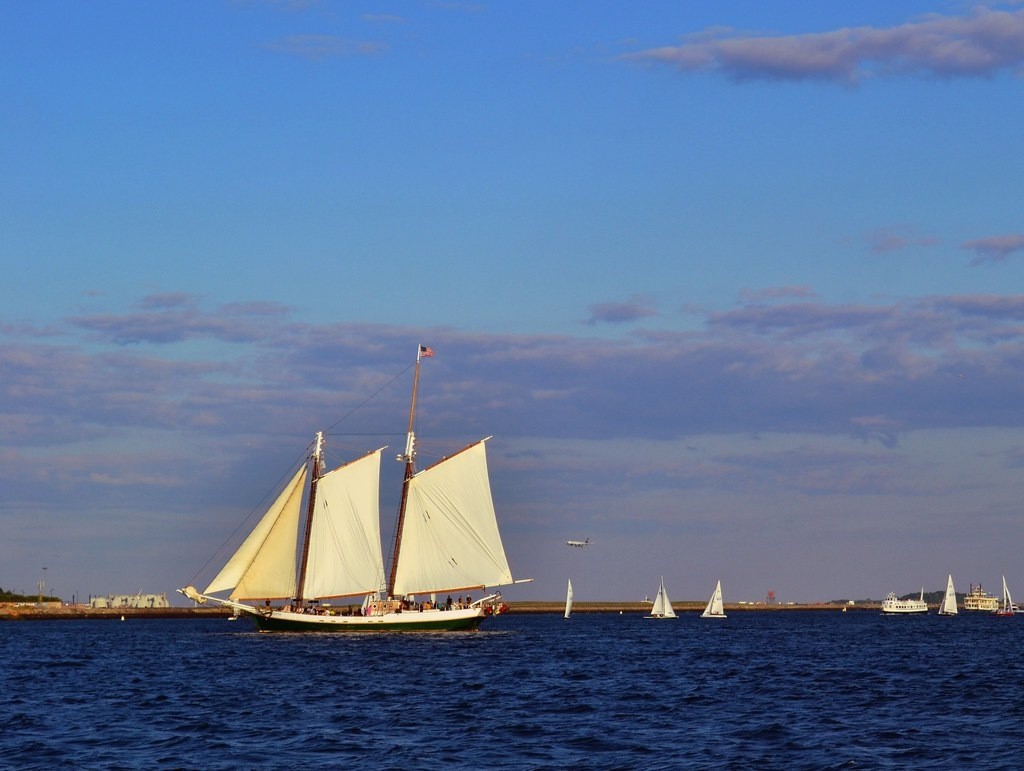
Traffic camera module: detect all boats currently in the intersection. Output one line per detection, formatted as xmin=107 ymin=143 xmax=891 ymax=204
xmin=1005 ymin=602 xmax=1024 ymax=612
xmin=964 ymin=583 xmax=999 ymax=611
xmin=881 ymin=587 xmax=929 ymax=612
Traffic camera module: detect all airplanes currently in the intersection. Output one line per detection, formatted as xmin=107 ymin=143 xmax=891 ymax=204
xmin=566 ymin=537 xmax=595 ymax=547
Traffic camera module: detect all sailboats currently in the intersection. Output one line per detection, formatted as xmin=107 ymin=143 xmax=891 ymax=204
xmin=563 ymin=580 xmax=574 ymax=619
xmin=700 ymin=579 xmax=728 ymax=618
xmin=643 ymin=574 xmax=680 ymax=619
xmin=176 ymin=344 xmax=533 ymax=633
xmin=939 ymin=574 xmax=958 ymax=615
xmin=997 ymin=575 xmax=1014 ymax=615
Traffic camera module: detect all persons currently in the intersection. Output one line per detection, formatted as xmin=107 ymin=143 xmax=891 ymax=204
xmin=395 ymin=594 xmax=472 ymax=613
xmin=265 ymin=598 xmax=271 ymax=611
xmin=364 ymin=604 xmax=393 ymax=617
xmin=289 ymin=604 xmax=362 ymax=616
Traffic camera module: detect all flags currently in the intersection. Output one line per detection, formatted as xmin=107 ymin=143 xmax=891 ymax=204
xmin=419 ymin=344 xmax=435 ymax=358
